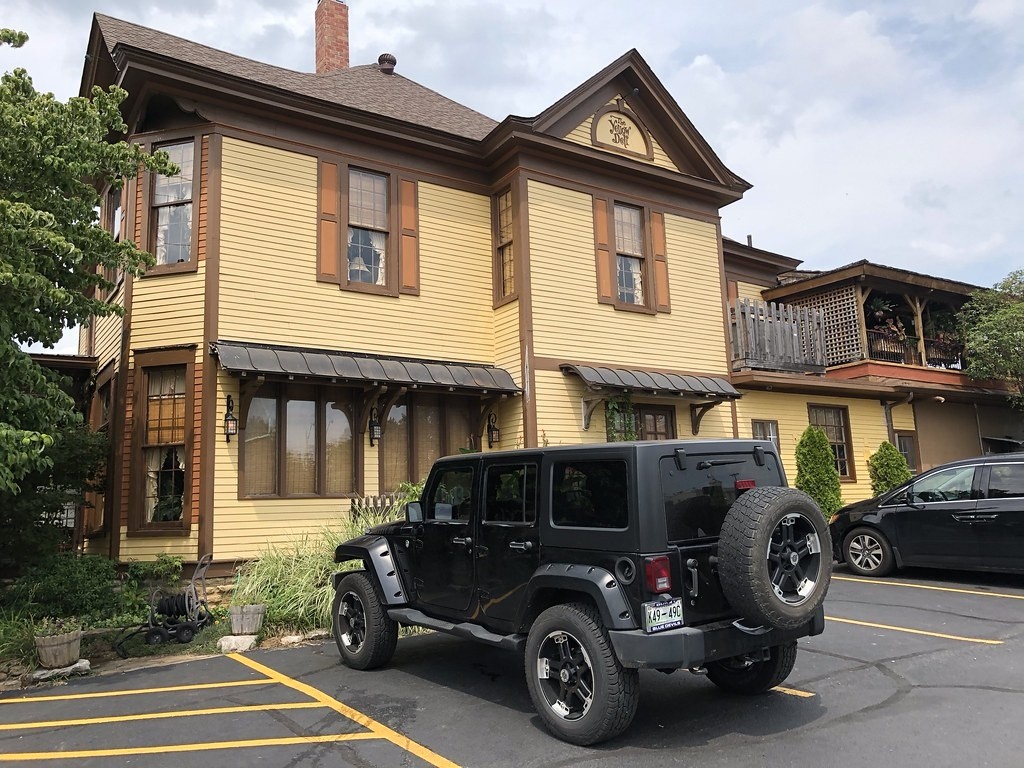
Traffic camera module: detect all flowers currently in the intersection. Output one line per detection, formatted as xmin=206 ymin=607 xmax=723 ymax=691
xmin=873 ymin=318 xmax=907 ymax=343
xmin=930 ymin=331 xmax=962 ymax=351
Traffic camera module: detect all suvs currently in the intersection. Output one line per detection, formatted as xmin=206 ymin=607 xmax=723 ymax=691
xmin=330 ymin=438 xmax=835 ymax=747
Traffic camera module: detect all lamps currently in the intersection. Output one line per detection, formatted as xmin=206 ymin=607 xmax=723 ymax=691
xmin=368 ymin=406 xmax=382 ymax=448
xmin=349 ymin=256 xmax=369 ymax=280
xmin=223 ymin=393 xmax=238 ymax=443
xmin=486 ymin=409 xmax=499 ymax=450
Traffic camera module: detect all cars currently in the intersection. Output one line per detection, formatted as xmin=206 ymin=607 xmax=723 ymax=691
xmin=828 ymin=452 xmax=1024 ymax=578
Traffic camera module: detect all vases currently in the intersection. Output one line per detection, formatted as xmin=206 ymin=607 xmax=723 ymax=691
xmin=926 ymin=347 xmax=955 ymax=360
xmin=871 ymin=338 xmax=905 ymax=353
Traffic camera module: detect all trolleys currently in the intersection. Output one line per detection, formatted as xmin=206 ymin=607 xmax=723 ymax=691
xmin=145 ymin=552 xmax=216 ymax=647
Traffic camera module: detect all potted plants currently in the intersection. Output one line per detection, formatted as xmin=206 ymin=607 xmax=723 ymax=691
xmin=34 ymin=615 xmax=82 ymax=668
xmin=227 ymin=592 xmax=268 ymax=636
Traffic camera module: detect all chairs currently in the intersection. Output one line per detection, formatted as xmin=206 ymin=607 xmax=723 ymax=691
xmin=505 ymin=472 xmax=538 ymax=521
